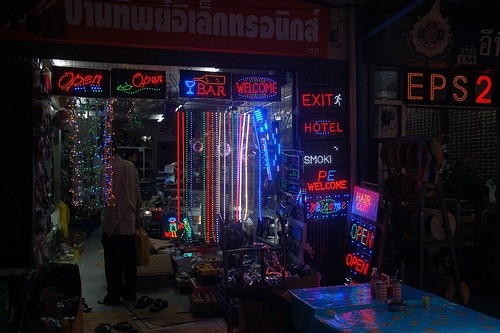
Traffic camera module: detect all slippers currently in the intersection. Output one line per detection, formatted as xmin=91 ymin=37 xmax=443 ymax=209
xmin=95 ymin=323 xmax=112 ymax=333
xmin=113 ymin=321 xmax=138 ymax=333
xmin=149 ymin=299 xmax=168 ymax=312
xmin=134 ymin=296 xmax=154 ymax=309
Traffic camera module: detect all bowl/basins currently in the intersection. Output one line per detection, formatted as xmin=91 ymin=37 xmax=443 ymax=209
xmin=386 ymin=301 xmax=406 ymax=311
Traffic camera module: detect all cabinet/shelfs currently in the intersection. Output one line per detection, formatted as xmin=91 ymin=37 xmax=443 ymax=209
xmin=116 ymin=147 xmax=158 ymax=180
xmin=356 ymin=67 xmax=407 ymax=260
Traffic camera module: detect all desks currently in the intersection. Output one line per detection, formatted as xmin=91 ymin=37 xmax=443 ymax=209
xmin=282 ymin=283 xmax=500 ymax=333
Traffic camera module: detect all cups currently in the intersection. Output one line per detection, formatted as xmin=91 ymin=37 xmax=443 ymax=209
xmin=375 ymin=282 xmax=387 ymax=300
xmin=391 ymin=279 xmax=402 ymax=300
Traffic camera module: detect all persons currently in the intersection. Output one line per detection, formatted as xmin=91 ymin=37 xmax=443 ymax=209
xmin=97 ymin=141 xmax=139 ymax=306
xmin=127 ymin=151 xmax=138 ymax=164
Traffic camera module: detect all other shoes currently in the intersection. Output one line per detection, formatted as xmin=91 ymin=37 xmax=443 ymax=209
xmin=97 ymin=298 xmax=104 ymax=304
xmin=82 ymin=298 xmax=92 ymax=311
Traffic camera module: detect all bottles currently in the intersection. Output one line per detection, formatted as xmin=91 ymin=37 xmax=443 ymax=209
xmin=371 ymin=267 xmax=379 ymax=282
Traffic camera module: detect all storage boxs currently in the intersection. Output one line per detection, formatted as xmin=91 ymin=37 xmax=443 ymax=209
xmin=195 ymin=270 xmax=218 ymax=285
xmin=73 ymin=217 xmax=100 ymax=238
xmin=21 ymin=263 xmax=84 ymax=333
xmin=137 ymin=253 xmax=174 ymax=289
xmin=188 ymin=278 xmax=218 ymax=292
xmin=286 ymin=261 xmax=320 ymax=288
xmin=191 ymin=292 xmax=220 ymax=320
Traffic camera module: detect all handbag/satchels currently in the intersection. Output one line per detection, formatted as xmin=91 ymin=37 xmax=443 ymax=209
xmin=136 ymin=226 xmax=149 ymax=267
